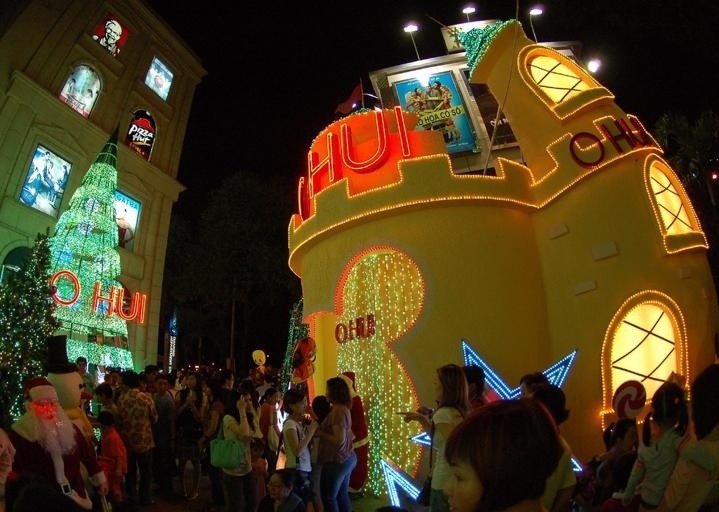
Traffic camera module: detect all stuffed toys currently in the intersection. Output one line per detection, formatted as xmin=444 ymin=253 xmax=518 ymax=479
xmin=43 ymin=334 xmax=97 ymax=448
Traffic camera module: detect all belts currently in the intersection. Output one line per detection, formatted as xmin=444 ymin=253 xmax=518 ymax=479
xmin=57 ymin=483 xmax=71 ymax=494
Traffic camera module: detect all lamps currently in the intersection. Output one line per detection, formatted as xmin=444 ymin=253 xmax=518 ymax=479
xmin=529 ymin=4 xmax=544 ymax=41
xmin=402 ymin=21 xmax=422 ymax=60
xmin=462 ymin=4 xmax=478 ymax=23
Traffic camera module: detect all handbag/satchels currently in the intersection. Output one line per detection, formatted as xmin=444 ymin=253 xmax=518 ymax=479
xmin=270 ymin=466 xmax=316 ymax=502
xmin=416 ymin=473 xmax=433 ymax=507
xmin=208 ymin=419 xmax=245 ymax=469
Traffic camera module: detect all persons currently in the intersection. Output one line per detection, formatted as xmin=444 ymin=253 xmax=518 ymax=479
xmin=374 ymin=364 xmax=718 ymax=512
xmin=49 ymin=165 xmax=69 ymax=206
xmin=1 ymin=359 xmax=369 ymax=512
xmin=36 ymin=152 xmax=54 ymax=192
xmin=79 ymin=89 xmax=93 ymax=110
xmin=91 ymin=19 xmax=122 ymax=58
xmin=61 ymin=78 xmax=76 ymax=100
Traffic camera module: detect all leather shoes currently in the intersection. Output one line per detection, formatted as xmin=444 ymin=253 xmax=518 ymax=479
xmin=181 ymin=492 xmax=198 ymax=501
